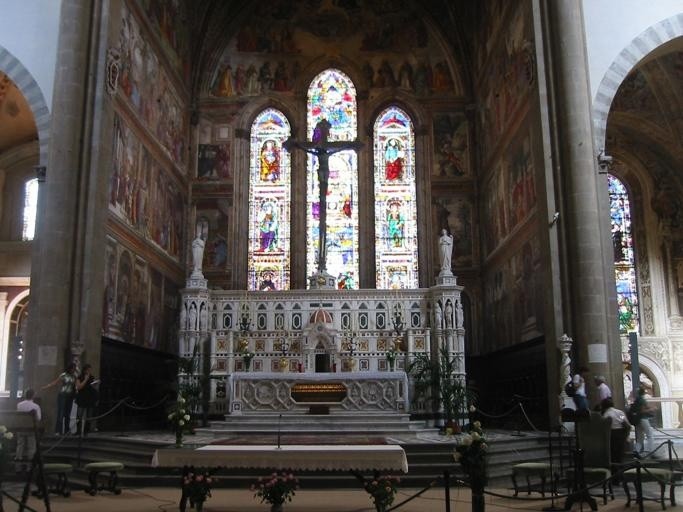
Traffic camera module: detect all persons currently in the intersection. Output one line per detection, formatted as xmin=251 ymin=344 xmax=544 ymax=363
xmin=12 ymin=388 xmax=42 ymax=473
xmin=571 ymin=363 xmax=591 ymax=412
xmin=71 ymin=365 xmax=97 ymax=435
xmin=594 ymin=375 xmax=612 ymax=402
xmin=602 ymin=398 xmax=629 ymax=461
xmin=633 ymin=388 xmax=658 ymax=459
xmin=33 ymin=362 xmax=85 ymax=433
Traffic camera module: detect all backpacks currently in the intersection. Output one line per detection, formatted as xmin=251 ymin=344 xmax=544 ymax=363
xmin=625 ymin=396 xmax=644 ymax=426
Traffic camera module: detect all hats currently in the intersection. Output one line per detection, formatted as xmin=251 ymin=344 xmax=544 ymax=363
xmin=593 ymin=375 xmax=605 ymax=384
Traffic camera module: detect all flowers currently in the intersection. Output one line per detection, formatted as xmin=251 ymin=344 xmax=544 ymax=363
xmin=451 ymin=402 xmax=490 ymax=495
xmin=168 ymin=391 xmax=190 ymax=445
xmin=249 ymin=471 xmax=299 ymax=506
xmin=181 ymin=471 xmax=217 ymax=505
xmin=362 ymin=473 xmax=400 ymax=507
xmin=0 ymin=425 xmax=14 ymax=449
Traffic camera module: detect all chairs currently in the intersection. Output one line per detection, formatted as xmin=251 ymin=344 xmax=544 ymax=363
xmin=512 ymin=425 xmax=683 ymax=512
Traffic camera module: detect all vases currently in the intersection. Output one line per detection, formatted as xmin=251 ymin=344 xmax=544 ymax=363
xmin=375 ymin=498 xmax=390 ymax=512
xmin=270 ymin=496 xmax=283 ymax=512
xmin=472 ymin=487 xmax=484 ymax=512
xmin=176 ymin=416 xmax=184 ymax=448
xmin=189 ymin=497 xmax=207 ymax=512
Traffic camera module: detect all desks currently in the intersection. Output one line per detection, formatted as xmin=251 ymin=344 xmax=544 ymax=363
xmin=150 ymin=442 xmax=409 ymax=512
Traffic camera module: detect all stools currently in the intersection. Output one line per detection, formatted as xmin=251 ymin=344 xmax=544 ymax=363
xmin=85 ymin=461 xmax=124 ymax=496
xmin=30 ymin=464 xmax=72 ymax=499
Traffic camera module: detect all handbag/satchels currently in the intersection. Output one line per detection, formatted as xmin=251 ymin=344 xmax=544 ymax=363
xmin=564 ymin=380 xmax=577 ymax=398
xmin=622 ymin=421 xmax=631 ymax=433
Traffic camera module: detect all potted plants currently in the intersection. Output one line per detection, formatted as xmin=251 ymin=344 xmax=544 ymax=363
xmin=157 ymin=341 xmax=206 ymax=435
xmin=405 ymin=341 xmax=478 ymax=435
